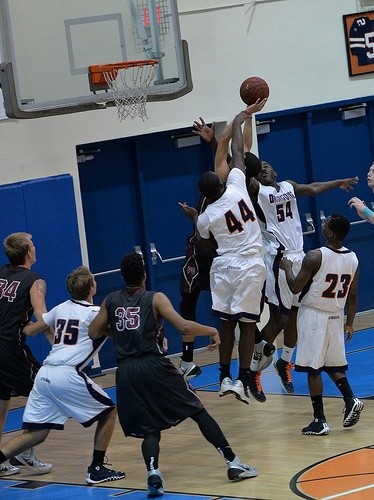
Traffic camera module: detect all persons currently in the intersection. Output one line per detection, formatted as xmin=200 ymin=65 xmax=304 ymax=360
xmin=0 ymin=267 xmax=126 ymax=484
xmin=88 ymin=252 xmax=261 ymax=497
xmin=0 ymin=232 xmax=55 ymax=474
xmin=348 ymin=162 xmax=374 ymax=227
xmin=279 ymin=214 xmax=364 ymax=436
xmin=177 ymin=97 xmax=359 ymax=405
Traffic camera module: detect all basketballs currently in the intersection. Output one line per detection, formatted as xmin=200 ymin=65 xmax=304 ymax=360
xmin=239 ymin=76 xmax=270 ymax=105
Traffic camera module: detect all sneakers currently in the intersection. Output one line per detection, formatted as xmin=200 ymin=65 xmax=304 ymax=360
xmin=342 ymin=396 xmax=364 ymax=427
xmin=218 ymin=377 xmax=233 ymax=397
xmin=147 ymin=468 xmax=164 ymax=496
xmin=0 ymin=464 xmax=20 ymax=476
xmin=9 ymin=450 xmax=53 ymax=473
xmin=250 ymin=350 xmax=259 ymax=371
xmin=273 ymin=358 xmax=294 ymax=394
xmin=248 ymin=371 xmax=266 ymax=403
xmin=302 ymin=417 xmax=331 ymax=434
xmin=86 ymin=455 xmax=126 ymax=483
xmin=254 ymin=340 xmax=276 ymax=370
xmin=177 ymin=359 xmax=202 ymax=381
xmin=232 ymin=380 xmax=250 ymax=404
xmin=226 ymin=456 xmax=257 ymax=480
